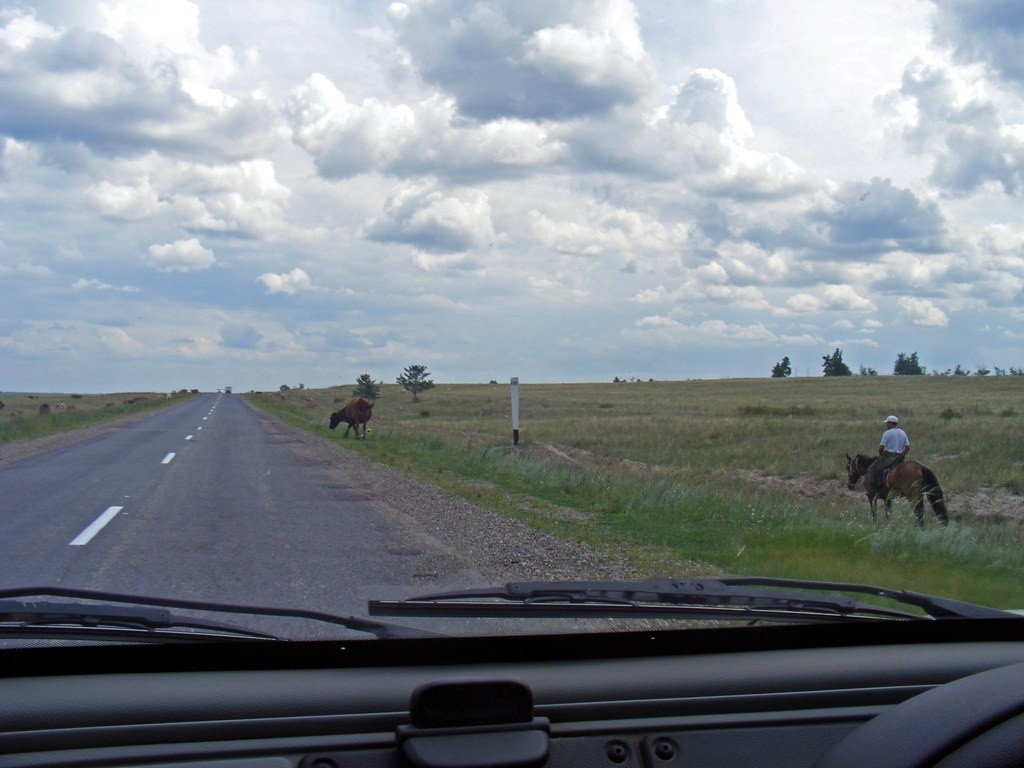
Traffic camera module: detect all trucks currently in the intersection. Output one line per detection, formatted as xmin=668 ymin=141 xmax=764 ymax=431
xmin=217 ymin=388 xmax=223 ymax=393
xmin=225 ymin=386 xmax=231 ymax=393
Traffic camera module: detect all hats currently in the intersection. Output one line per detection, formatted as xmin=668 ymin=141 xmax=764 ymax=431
xmin=884 ymin=416 xmax=898 ymax=423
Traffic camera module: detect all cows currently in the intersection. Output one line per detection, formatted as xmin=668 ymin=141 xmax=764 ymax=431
xmin=328 ymin=397 xmax=375 ymax=439
xmin=249 ymin=389 xmax=346 ymax=409
xmin=0 ymin=391 xmax=152 ymax=419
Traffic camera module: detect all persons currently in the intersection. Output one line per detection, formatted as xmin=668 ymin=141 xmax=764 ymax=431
xmin=865 ymin=416 xmax=910 ymax=496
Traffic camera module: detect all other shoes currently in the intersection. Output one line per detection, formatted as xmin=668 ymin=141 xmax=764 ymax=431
xmin=865 ymin=489 xmax=875 ymax=495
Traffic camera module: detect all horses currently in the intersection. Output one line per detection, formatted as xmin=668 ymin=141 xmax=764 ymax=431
xmin=845 ymin=452 xmax=949 ymax=530
